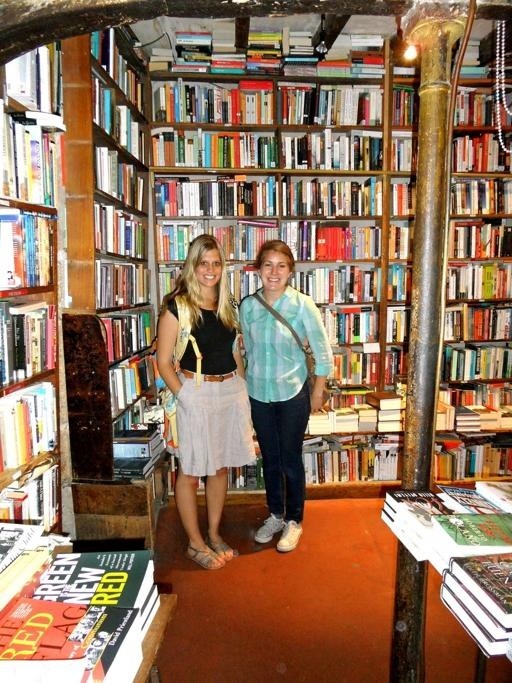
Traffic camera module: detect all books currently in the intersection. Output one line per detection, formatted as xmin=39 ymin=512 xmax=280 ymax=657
xmin=381 ymin=481 xmax=512 ymax=663
xmin=0 ymin=551 xmax=160 ymax=682
xmin=91 ymin=28 xmax=512 ymax=310
xmin=99 ymin=310 xmax=167 ymax=480
xmin=303 ymin=302 xmax=512 ymax=485
xmin=166 ymin=452 xmax=206 ymax=492
xmin=1 ymin=39 xmax=59 ymax=572
xmin=227 ymin=331 xmax=265 ymax=491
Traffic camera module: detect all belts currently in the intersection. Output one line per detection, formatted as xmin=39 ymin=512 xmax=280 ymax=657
xmin=181 ymin=368 xmax=236 ymax=381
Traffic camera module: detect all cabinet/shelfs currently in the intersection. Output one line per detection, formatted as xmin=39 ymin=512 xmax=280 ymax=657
xmin=164 ymin=423 xmax=512 ymax=509
xmin=369 ymin=62 xmax=512 ymax=419
xmin=61 ymin=14 xmax=155 ymax=316
xmin=59 ymin=308 xmax=162 ymax=493
xmin=65 ymin=446 xmax=174 ymax=566
xmin=147 ymin=60 xmax=388 ymax=409
xmin=2 ymin=27 xmax=69 ymax=547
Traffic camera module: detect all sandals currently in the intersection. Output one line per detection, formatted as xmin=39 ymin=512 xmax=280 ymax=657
xmin=185 ymin=535 xmax=233 ymax=568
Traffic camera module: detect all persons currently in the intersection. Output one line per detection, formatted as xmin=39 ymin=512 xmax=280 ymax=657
xmin=157 ymin=233 xmax=258 ymax=569
xmin=240 ymin=240 xmax=333 ymax=552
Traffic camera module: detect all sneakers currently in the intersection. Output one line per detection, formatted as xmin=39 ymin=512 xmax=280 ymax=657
xmin=255 ymin=512 xmax=284 ymax=542
xmin=277 ymin=519 xmax=303 ymax=551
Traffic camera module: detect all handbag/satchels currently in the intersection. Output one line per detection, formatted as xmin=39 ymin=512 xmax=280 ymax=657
xmin=159 ymin=386 xmax=178 ymax=448
xmin=305 ymin=352 xmax=330 ymax=406
